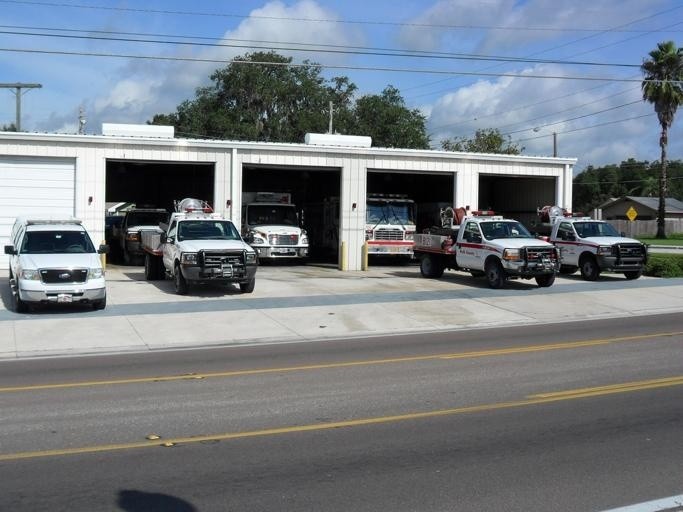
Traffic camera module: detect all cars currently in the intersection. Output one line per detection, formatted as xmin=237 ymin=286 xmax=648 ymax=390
xmin=4 ymin=216 xmax=110 ymax=311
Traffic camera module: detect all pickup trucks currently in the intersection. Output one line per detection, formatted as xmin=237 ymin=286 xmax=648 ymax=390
xmin=106 ymin=198 xmax=258 ymax=295
xmin=412 ymin=203 xmax=647 ymax=289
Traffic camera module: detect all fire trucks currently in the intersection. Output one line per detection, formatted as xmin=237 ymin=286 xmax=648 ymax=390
xmin=324 ymin=191 xmax=416 ymax=265
xmin=241 ymin=191 xmax=309 ymax=265
